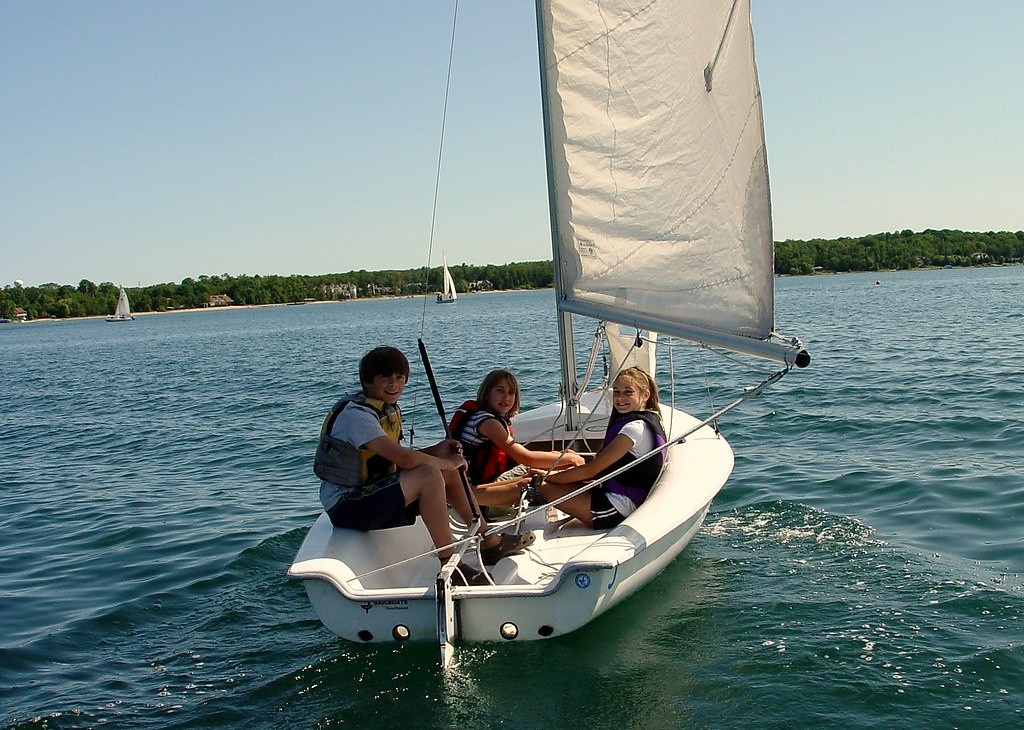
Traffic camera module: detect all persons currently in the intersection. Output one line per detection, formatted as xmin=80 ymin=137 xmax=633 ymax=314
xmin=445 ymin=366 xmax=668 ymax=530
xmin=313 ymin=346 xmax=536 ymax=586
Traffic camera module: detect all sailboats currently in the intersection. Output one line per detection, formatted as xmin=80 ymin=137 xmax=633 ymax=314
xmin=436 ymin=250 xmax=458 ymax=303
xmin=104 ymin=284 xmax=135 ymax=322
xmin=286 ymin=1 xmax=812 ymax=670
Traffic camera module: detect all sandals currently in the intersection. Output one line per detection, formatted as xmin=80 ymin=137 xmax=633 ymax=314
xmin=476 ymin=532 xmax=536 ymax=564
xmin=436 ymin=564 xmax=494 ymax=586
